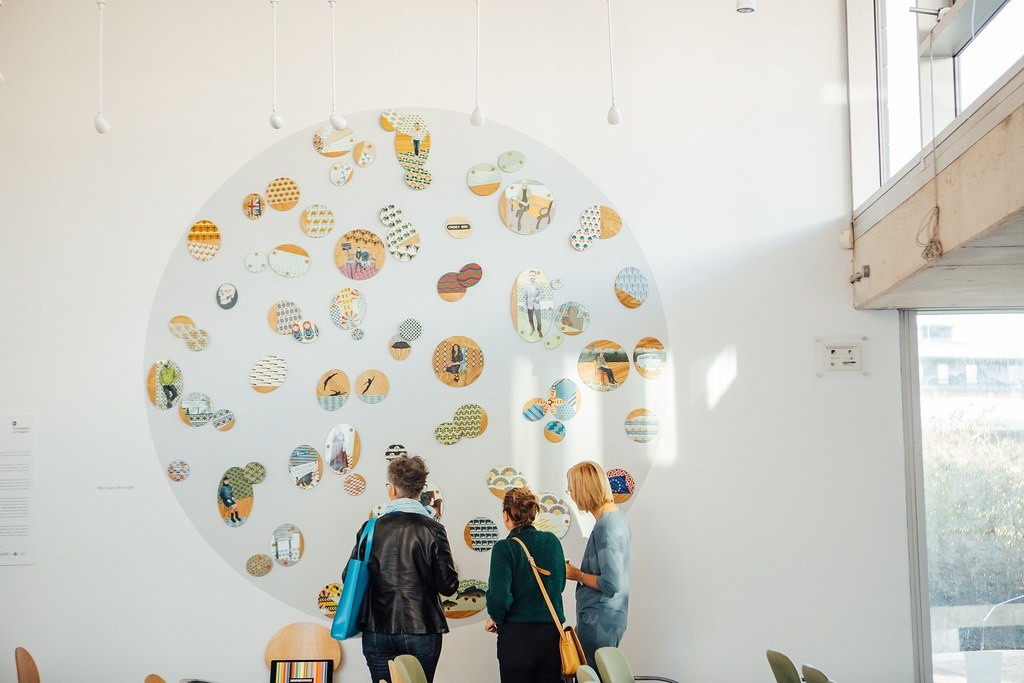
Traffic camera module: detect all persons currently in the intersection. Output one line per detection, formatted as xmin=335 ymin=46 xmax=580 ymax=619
xmin=565 ymin=461 xmax=630 ymax=682
xmin=597 ymin=350 xmax=618 ymax=384
xmin=525 ymin=269 xmax=545 ymax=339
xmin=446 ymin=344 xmax=463 ymax=382
xmin=515 ymin=179 xmax=531 ymax=231
xmin=354 ymin=246 xmax=369 ymax=273
xmin=411 ymin=122 xmax=422 ymax=158
xmin=220 ymin=477 xmax=242 ymax=523
xmin=341 ymin=457 xmax=460 ymax=683
xmin=159 ymin=359 xmax=179 ymax=410
xmin=485 ymin=487 xmax=565 ymax=683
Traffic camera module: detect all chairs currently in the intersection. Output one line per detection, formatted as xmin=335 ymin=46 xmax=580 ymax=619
xmin=801 ymin=663 xmax=837 ymax=683
xmin=577 ymin=665 xmax=601 ymax=683
xmin=595 ymin=647 xmax=678 ymax=683
xmin=15 ymin=646 xmax=40 ymax=683
xmin=387 ymin=655 xmax=428 ymax=682
xmin=766 ymin=649 xmax=801 ymax=683
xmin=264 ymin=622 xmax=342 ymax=671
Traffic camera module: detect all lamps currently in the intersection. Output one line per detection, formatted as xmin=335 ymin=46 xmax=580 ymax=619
xmin=269 ymin=0 xmax=283 ymax=129
xmin=93 ymin=1 xmax=112 ymax=133
xmin=469 ymin=0 xmax=485 ymax=126
xmin=607 ymin=0 xmax=622 ymax=124
xmin=329 ymin=0 xmax=346 ymax=130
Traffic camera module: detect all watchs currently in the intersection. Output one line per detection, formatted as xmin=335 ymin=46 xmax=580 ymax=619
xmin=578 ymin=572 xmax=584 ymax=585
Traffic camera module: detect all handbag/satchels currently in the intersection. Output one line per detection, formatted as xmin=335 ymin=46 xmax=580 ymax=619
xmin=558 ymin=627 xmax=587 ymax=675
xmin=330 ymin=518 xmax=376 ymax=641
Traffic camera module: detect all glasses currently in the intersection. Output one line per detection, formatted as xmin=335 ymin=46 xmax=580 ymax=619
xmin=385 ymin=482 xmax=391 ymax=490
xmin=565 ymin=487 xmax=574 ymax=495
